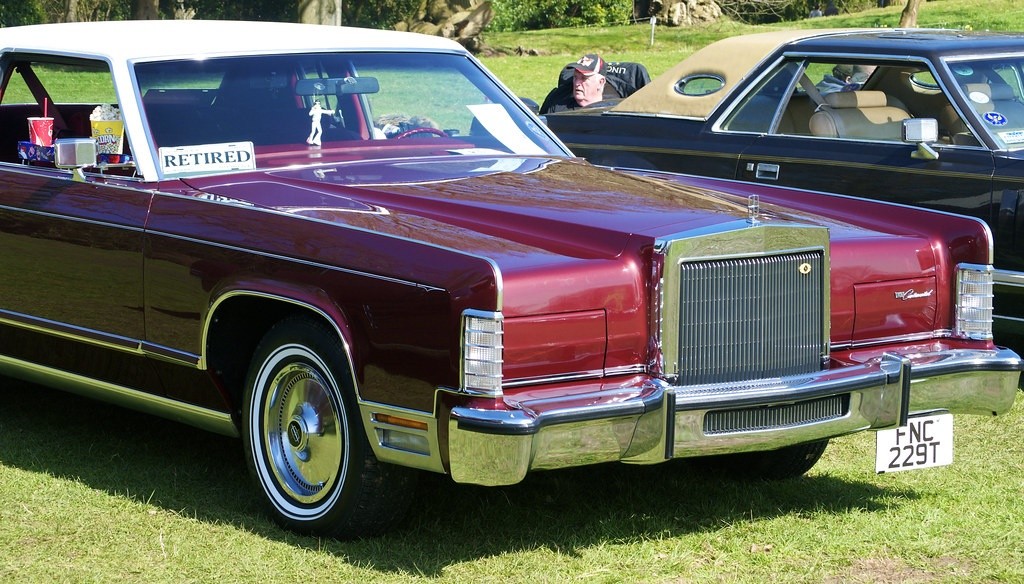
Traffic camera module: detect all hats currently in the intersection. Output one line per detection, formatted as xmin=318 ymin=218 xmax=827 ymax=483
xmin=566 ymin=54 xmax=608 ymax=77
xmin=835 ymin=64 xmax=865 ymax=77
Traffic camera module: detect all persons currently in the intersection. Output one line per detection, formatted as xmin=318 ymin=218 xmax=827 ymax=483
xmin=546 ymin=53 xmax=618 ymax=115
xmin=306 ymin=100 xmax=335 ymax=146
xmin=815 ymin=64 xmax=877 ymax=88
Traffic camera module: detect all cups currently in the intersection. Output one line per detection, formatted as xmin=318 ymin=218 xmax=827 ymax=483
xmin=91 ymin=119 xmax=125 ymax=164
xmin=27 ymin=117 xmax=54 ymax=146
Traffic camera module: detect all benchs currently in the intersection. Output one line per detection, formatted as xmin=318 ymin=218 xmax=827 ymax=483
xmin=731 ymin=94 xmax=916 ymax=135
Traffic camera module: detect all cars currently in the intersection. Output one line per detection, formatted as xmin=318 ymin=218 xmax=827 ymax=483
xmin=0 ymin=20 xmax=1023 ymax=540
xmin=542 ymin=28 xmax=1024 ymax=334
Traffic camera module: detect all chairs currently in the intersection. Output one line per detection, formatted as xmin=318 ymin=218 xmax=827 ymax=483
xmin=809 ymin=90 xmax=912 ymax=142
xmin=945 ymin=81 xmax=1024 ymax=134
xmin=540 ymin=62 xmax=651 ymax=115
